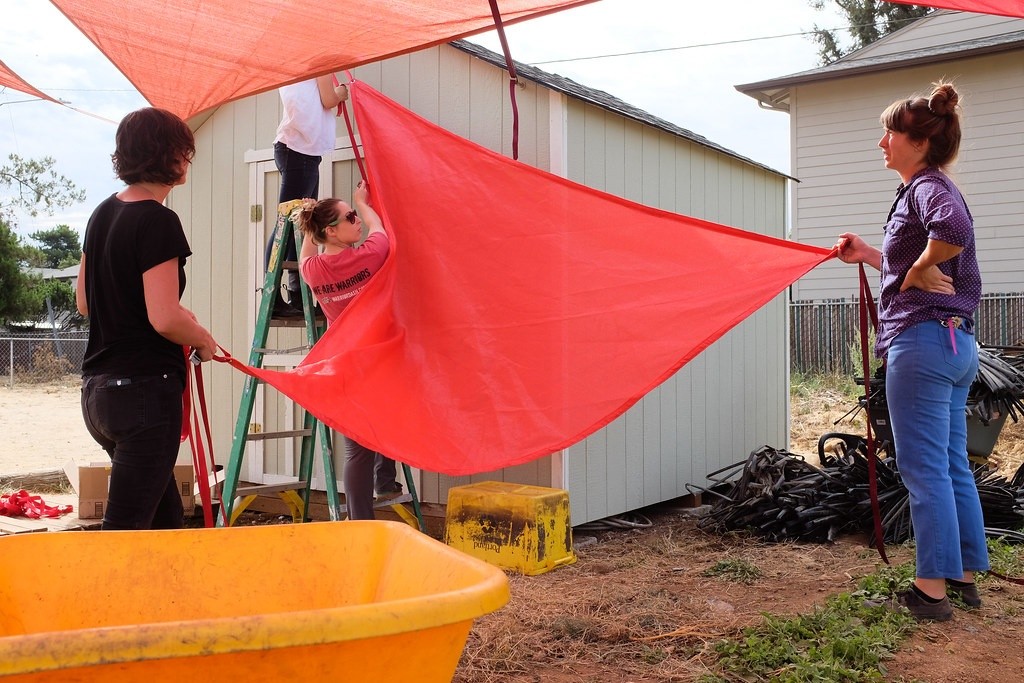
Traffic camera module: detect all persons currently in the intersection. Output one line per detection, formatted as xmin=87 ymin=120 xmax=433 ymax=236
xmin=296 ymin=181 xmax=404 ymax=522
xmin=75 ymin=108 xmax=218 ymax=532
xmin=258 ymin=74 xmax=363 ymax=323
xmin=831 ymin=81 xmax=994 ymax=622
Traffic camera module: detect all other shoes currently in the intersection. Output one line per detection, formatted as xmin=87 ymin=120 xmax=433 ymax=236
xmin=377 ymin=480 xmax=405 ymax=502
xmin=940 ymin=576 xmax=981 ymax=611
xmin=891 ymin=585 xmax=955 ymax=623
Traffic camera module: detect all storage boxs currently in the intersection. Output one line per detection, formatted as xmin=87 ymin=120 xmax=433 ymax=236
xmin=443 ymin=481 xmax=579 ymax=577
xmin=62 ymin=461 xmax=227 ymax=521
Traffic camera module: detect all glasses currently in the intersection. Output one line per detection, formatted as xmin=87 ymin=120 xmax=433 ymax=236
xmin=320 ymin=208 xmax=359 ymax=233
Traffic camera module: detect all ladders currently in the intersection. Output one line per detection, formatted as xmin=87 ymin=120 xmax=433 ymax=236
xmin=215 ymin=200 xmax=427 ymax=534
